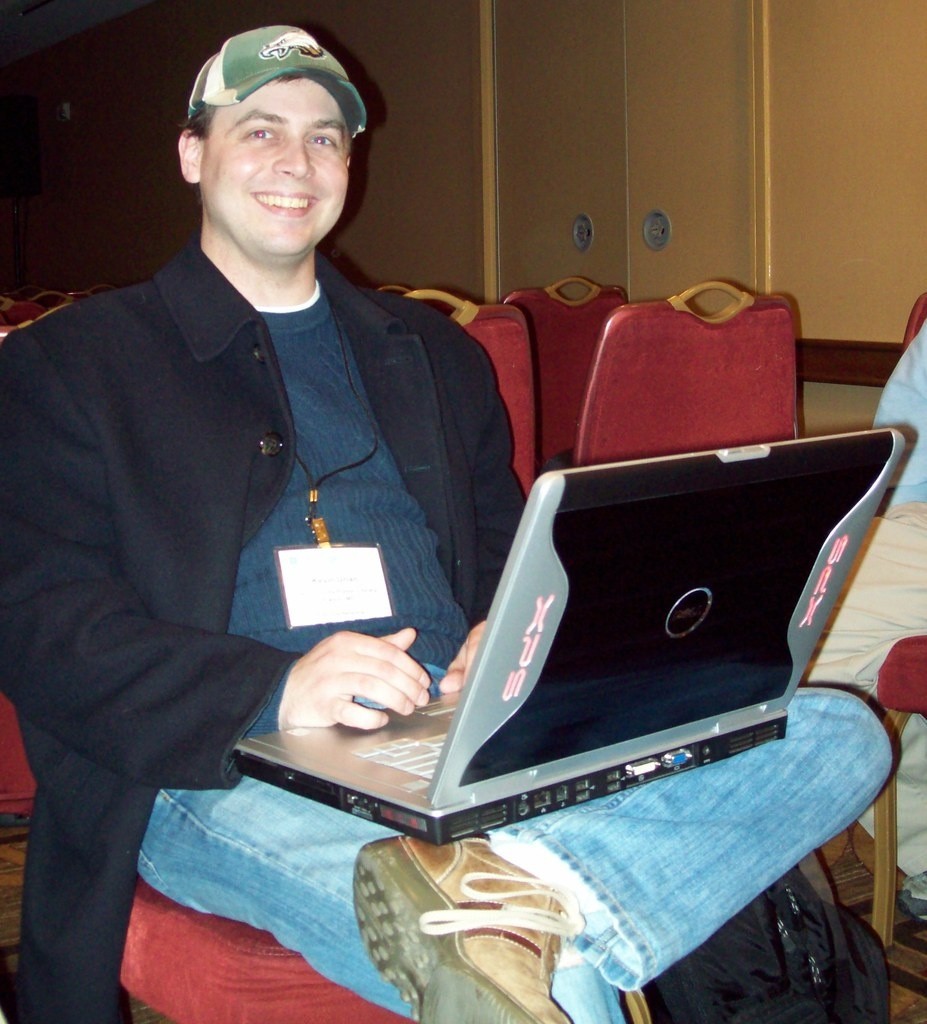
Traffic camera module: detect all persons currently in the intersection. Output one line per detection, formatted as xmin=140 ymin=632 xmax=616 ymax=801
xmin=872 ymin=319 xmax=927 ymax=514
xmin=2 ymin=23 xmax=893 ymax=1024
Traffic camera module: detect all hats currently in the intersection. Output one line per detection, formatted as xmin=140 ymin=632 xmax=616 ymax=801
xmin=186 ymin=23 xmax=369 ymax=140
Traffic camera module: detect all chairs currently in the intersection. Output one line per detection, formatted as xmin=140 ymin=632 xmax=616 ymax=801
xmin=400 ymin=289 xmax=535 ymax=498
xmin=500 ymin=277 xmax=626 ymax=468
xmin=0 ymin=285 xmax=118 ymax=349
xmin=873 ymin=635 xmax=927 ymax=950
xmin=119 ymin=881 xmax=420 ymax=1024
xmin=573 ymin=279 xmax=798 ymax=471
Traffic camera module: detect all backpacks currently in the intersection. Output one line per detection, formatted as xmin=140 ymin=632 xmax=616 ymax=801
xmin=642 ymin=864 xmax=891 ymax=1024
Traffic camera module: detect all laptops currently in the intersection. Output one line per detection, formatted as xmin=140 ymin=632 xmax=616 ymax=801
xmin=243 ymin=420 xmax=904 ymax=847
